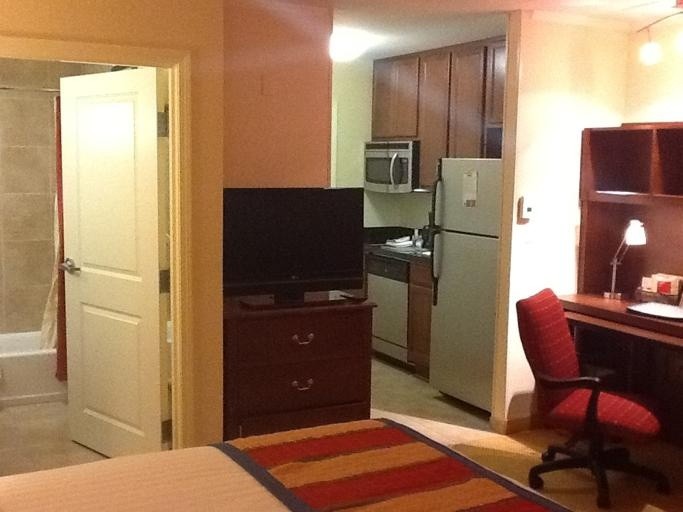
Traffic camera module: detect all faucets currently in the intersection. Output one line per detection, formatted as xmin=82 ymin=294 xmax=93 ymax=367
xmin=412 ymin=228 xmax=419 ymax=248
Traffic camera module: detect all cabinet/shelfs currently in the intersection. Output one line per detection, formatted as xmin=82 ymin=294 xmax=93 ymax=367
xmin=373 ymin=53 xmax=420 ymax=141
xmin=223 ymin=290 xmax=378 ymax=441
xmin=420 ymin=39 xmax=485 ymax=187
xmin=408 ymin=263 xmax=433 ymax=379
xmin=557 ymin=122 xmax=683 ymax=446
xmin=486 ymin=35 xmax=506 ymax=128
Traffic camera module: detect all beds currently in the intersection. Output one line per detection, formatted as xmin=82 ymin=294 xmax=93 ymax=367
xmin=0 ymin=419 xmax=572 ymax=512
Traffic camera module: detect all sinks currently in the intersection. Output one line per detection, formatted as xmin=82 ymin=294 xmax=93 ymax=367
xmin=369 ymin=243 xmax=432 ymax=262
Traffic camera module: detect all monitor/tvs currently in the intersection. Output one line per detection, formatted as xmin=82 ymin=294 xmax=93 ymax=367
xmin=223 ymin=187 xmax=365 ymax=306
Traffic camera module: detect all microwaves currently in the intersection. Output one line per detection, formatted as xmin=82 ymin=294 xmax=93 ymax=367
xmin=365 ymin=141 xmax=415 ymax=194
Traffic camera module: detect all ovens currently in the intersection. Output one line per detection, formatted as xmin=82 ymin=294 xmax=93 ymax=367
xmin=366 ymin=253 xmax=409 ymax=347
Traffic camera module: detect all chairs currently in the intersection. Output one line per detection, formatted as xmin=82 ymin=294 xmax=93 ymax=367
xmin=516 ymin=288 xmax=670 ymax=508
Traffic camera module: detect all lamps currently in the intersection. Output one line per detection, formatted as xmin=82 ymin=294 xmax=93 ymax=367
xmin=637 ymin=11 xmax=683 ymax=65
xmin=604 ymin=219 xmax=647 ymax=300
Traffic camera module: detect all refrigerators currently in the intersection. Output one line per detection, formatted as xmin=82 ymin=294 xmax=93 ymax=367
xmin=427 ymin=157 xmax=504 ymax=413
